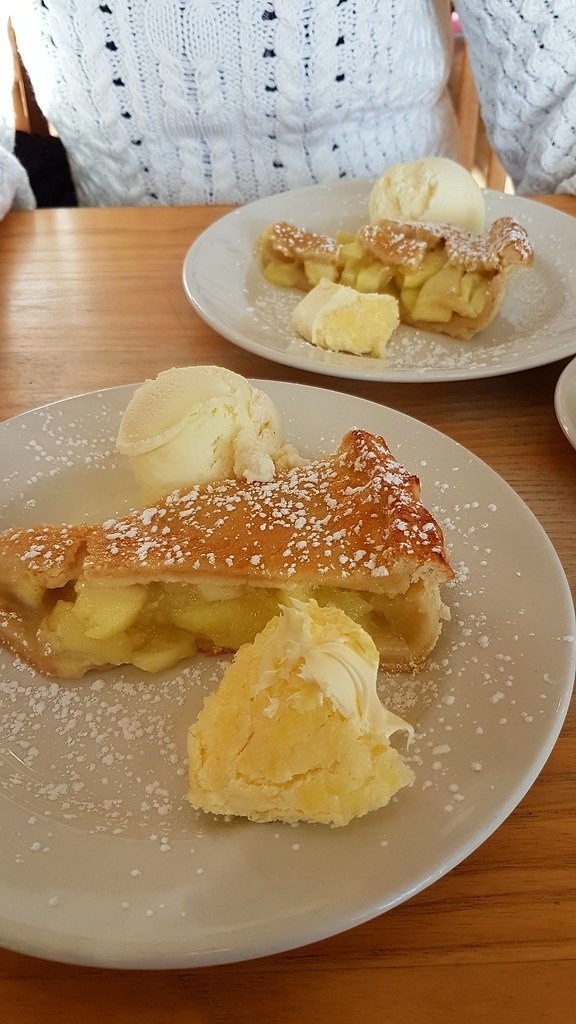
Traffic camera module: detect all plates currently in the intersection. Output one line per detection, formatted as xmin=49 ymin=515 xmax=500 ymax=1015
xmin=0 ymin=381 xmax=576 ymax=969
xmin=181 ymin=181 xmax=576 ymax=381
xmin=555 ymin=357 xmax=576 ymax=449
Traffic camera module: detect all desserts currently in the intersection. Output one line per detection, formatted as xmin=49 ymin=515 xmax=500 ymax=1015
xmin=0 ymin=363 xmax=455 ymax=827
xmin=263 ymin=153 xmax=535 ymax=357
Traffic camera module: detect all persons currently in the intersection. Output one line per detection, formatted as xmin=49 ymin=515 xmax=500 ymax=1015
xmin=0 ymin=0 xmax=576 ymax=223
